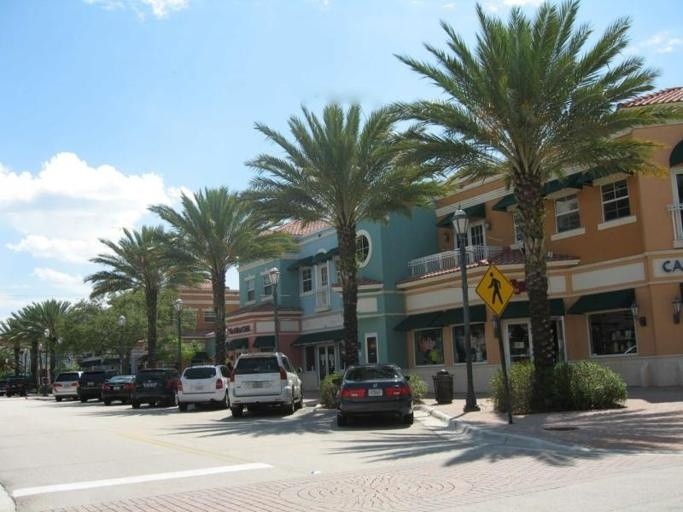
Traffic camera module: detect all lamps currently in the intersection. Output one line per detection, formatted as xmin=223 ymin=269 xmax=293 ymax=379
xmin=631 ymin=304 xmax=646 ymax=327
xmin=671 ymin=296 xmax=681 ymax=325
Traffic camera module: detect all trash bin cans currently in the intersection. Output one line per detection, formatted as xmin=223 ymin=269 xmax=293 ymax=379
xmin=432 ymin=368 xmax=454 ymax=404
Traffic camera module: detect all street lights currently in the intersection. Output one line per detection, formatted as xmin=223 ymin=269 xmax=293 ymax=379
xmin=118 ymin=314 xmax=125 ymax=375
xmin=43 ymin=328 xmax=49 ymax=396
xmin=451 ymin=204 xmax=481 ymax=412
xmin=268 ymin=265 xmax=280 ymax=351
xmin=172 ymin=298 xmax=183 ymax=376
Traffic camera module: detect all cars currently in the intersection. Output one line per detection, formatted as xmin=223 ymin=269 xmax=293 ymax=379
xmin=131 ymin=368 xmax=178 ymax=409
xmin=100 ymin=374 xmax=135 ymax=404
xmin=331 ymin=363 xmax=413 ymax=426
xmin=76 ymin=368 xmax=116 ymax=402
xmin=6 ymin=377 xmax=27 ymax=397
xmin=51 ymin=371 xmax=84 ymax=401
xmin=176 ymin=363 xmax=230 ymax=411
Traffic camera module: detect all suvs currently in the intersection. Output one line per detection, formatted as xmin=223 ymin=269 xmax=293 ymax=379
xmin=227 ymin=351 xmax=303 ymax=417
